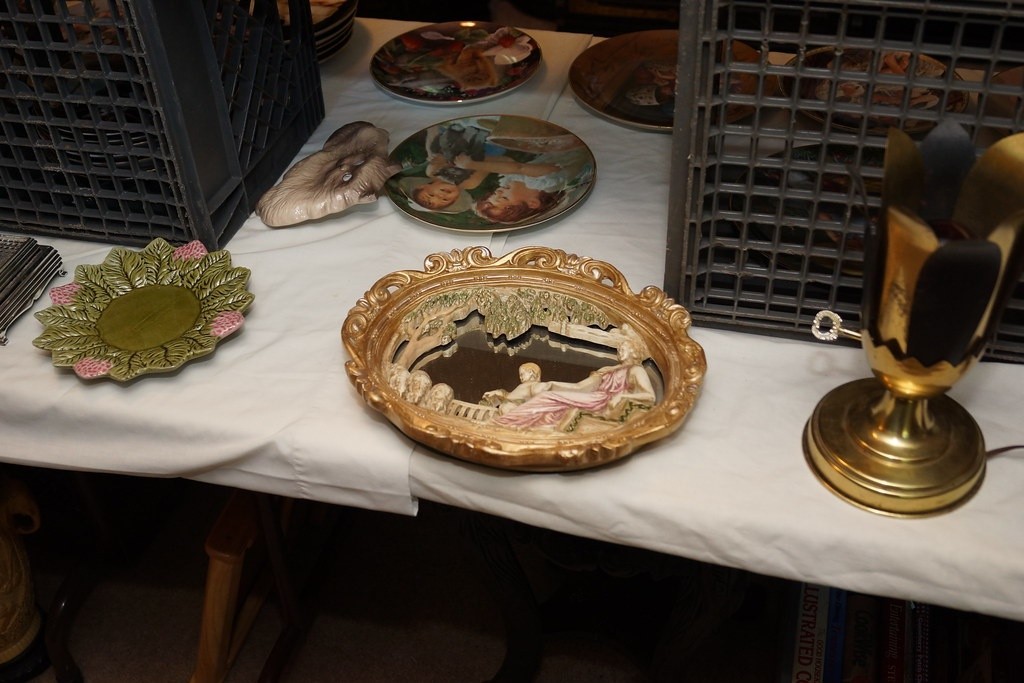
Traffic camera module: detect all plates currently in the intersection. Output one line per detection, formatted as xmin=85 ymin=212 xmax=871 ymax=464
xmin=978 ymin=66 xmax=1024 ymax=139
xmin=34 ymin=237 xmax=255 ymax=383
xmin=29 ymin=0 xmax=357 ymax=183
xmin=369 ymin=21 xmax=542 ymax=106
xmin=778 ymin=46 xmax=970 ymax=134
xmin=569 ymin=27 xmax=773 ymax=133
xmin=383 ymin=114 xmax=596 ymax=232
xmin=728 ymin=144 xmax=885 ymax=287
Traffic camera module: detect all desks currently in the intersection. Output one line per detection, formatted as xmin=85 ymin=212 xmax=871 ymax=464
xmin=0 ymin=19 xmax=1023 ymax=682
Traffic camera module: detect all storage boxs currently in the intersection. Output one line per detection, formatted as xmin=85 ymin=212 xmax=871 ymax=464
xmin=664 ymin=0 xmax=1023 ymax=366
xmin=0 ymin=0 xmax=326 ymax=253
